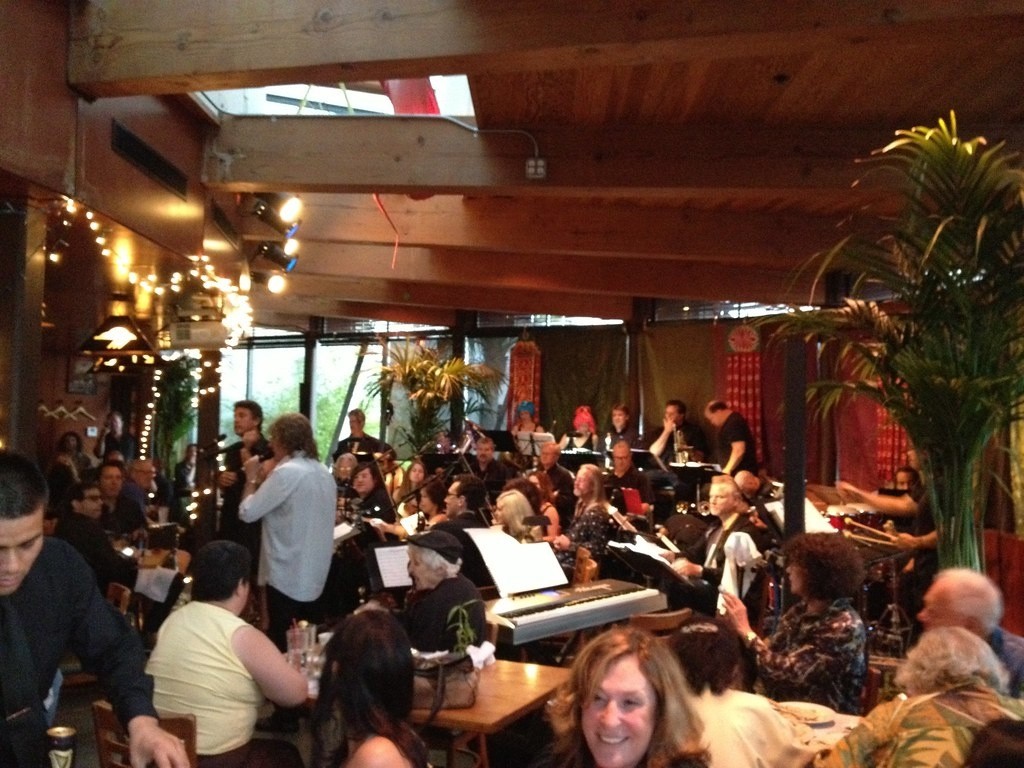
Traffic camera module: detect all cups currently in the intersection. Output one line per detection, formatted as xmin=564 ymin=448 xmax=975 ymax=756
xmin=286 ymin=628 xmax=309 ymax=677
xmin=291 ymin=624 xmax=317 ymax=665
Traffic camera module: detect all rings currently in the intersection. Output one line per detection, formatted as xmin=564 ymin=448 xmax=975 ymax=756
xmin=180 ymin=740 xmax=185 ymax=744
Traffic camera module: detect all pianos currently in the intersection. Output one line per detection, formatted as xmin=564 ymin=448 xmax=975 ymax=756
xmin=483 ymin=578 xmax=668 ymax=647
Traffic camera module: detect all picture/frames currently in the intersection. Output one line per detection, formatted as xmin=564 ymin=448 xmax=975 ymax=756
xmin=65 ymin=351 xmax=98 ymax=396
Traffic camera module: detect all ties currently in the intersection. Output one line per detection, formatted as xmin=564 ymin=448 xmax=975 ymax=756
xmin=0 ymin=598 xmax=51 ymax=768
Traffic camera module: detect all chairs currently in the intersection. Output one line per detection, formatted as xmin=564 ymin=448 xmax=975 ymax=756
xmin=58 ymin=581 xmax=133 ymax=699
xmin=92 ymin=699 xmax=197 ymax=768
xmin=630 ymin=605 xmax=692 ymax=640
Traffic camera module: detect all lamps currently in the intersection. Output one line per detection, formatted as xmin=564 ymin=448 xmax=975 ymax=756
xmin=250 ymin=270 xmax=286 ymax=294
xmin=235 ymin=193 xmax=301 ymax=239
xmin=251 ymin=192 xmax=303 ymax=223
xmin=254 ymin=240 xmax=299 ymax=272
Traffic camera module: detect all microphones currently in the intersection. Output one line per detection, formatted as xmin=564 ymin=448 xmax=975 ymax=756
xmin=465 ymin=418 xmax=486 ymax=440
xmin=198 ymin=434 xmax=227 ymax=453
xmin=401 ymin=428 xmax=421 ymax=450
xmin=239 ymin=451 xmax=274 ymax=473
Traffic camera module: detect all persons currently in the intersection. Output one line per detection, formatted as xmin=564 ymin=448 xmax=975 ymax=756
xmin=0 ymin=399 xmax=1024 ymax=768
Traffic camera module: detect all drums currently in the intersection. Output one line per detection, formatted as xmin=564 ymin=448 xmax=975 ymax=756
xmin=826 ymin=506 xmax=861 ymax=531
xmin=848 ymin=502 xmax=886 ymax=534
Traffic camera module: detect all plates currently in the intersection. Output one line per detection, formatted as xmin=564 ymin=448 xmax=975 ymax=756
xmin=781 ymin=701 xmax=835 ymax=725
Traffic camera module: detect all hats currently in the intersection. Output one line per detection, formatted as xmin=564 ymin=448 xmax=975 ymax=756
xmin=402 ymin=530 xmax=475 ymax=564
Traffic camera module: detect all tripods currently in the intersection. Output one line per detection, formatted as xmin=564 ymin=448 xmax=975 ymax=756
xmin=866 ymin=548 xmax=916 ymax=659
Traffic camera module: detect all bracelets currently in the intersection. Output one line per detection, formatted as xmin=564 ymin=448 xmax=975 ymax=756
xmin=745 ymin=632 xmax=757 ymax=645
xmin=246 ymin=480 xmax=256 ymax=484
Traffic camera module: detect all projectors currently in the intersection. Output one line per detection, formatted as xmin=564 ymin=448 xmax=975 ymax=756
xmin=169 ymin=322 xmax=231 ymax=348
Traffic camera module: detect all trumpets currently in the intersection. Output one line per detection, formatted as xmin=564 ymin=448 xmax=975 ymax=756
xmin=673 ymin=422 xmax=688 ymax=463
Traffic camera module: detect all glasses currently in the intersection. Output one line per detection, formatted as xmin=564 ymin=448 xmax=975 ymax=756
xmin=613 ymin=454 xmax=629 ymax=460
xmin=85 ymin=495 xmax=103 ymax=502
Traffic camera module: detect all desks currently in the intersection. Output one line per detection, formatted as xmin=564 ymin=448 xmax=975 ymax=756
xmin=813 ymin=713 xmax=862 ymax=747
xmin=280 ymin=642 xmax=572 ymax=768
